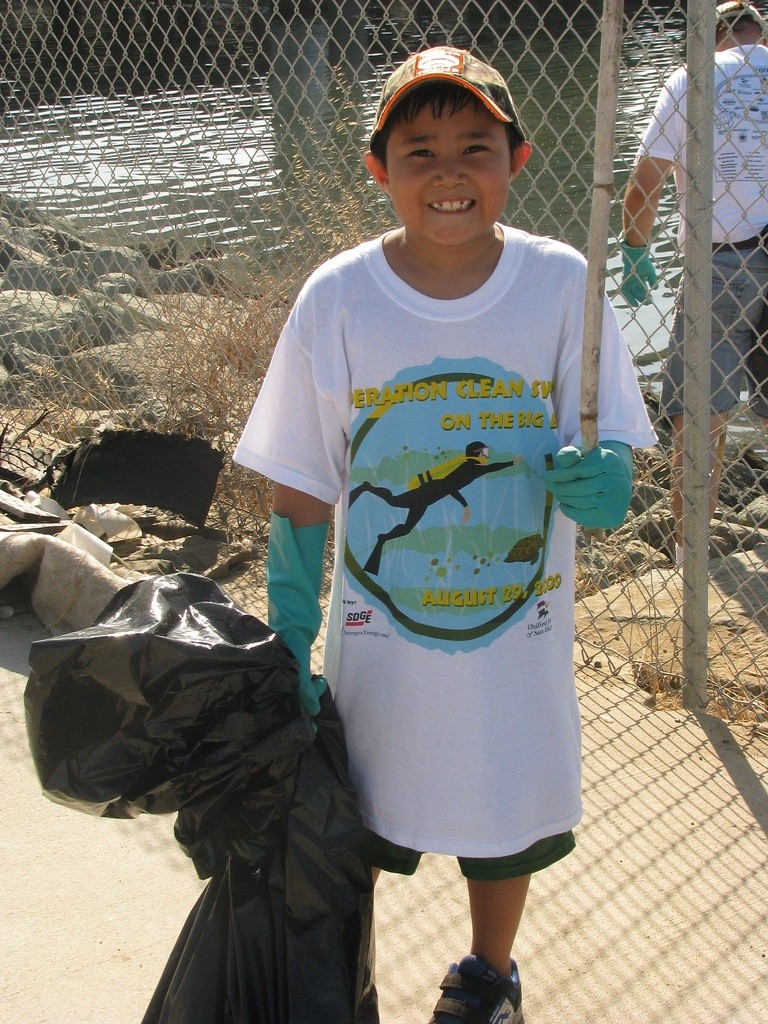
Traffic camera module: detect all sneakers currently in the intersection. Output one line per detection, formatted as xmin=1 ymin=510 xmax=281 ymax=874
xmin=426 ymin=954 xmax=525 ymax=1024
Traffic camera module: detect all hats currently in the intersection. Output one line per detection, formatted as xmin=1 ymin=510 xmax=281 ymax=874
xmin=715 ymin=1 xmax=768 ymax=39
xmin=370 ymin=46 xmax=527 ymax=148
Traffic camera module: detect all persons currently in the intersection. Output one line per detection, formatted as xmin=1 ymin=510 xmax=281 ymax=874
xmin=231 ymin=47 xmax=658 ymax=1024
xmin=621 ymin=0 xmax=768 ymax=566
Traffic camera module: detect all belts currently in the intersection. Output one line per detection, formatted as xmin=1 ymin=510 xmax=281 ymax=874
xmin=712 ymin=224 xmax=768 ymax=252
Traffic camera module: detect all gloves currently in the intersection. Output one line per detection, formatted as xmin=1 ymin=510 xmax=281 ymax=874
xmin=621 ymin=241 xmax=659 ymax=307
xmin=542 ymin=439 xmax=632 ymax=528
xmin=266 ymin=512 xmax=328 ymax=735
